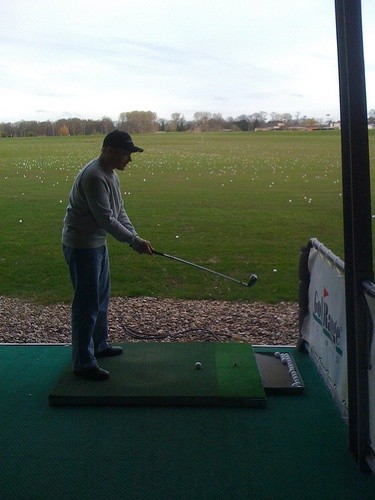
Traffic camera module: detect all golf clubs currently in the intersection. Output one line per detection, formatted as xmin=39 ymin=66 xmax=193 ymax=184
xmin=150 ymin=247 xmax=258 ymax=288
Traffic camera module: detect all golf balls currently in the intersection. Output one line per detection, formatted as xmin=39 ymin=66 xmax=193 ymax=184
xmin=194 ymin=361 xmax=202 ymax=370
xmin=274 ymin=350 xmax=303 ymax=388
xmin=0 ymin=119 xmax=375 ymax=275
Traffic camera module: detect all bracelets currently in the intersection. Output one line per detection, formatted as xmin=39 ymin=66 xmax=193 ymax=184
xmin=129 ymin=233 xmax=136 ymax=248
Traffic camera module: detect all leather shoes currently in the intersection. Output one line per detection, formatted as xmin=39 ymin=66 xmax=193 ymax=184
xmin=73 ymin=346 xmax=123 ymax=381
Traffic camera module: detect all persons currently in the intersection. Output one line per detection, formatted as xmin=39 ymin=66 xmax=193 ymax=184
xmin=62 ymin=130 xmax=154 ymax=384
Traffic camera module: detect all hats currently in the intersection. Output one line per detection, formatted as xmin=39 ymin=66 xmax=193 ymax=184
xmin=103 ymin=129 xmax=144 ymax=152
